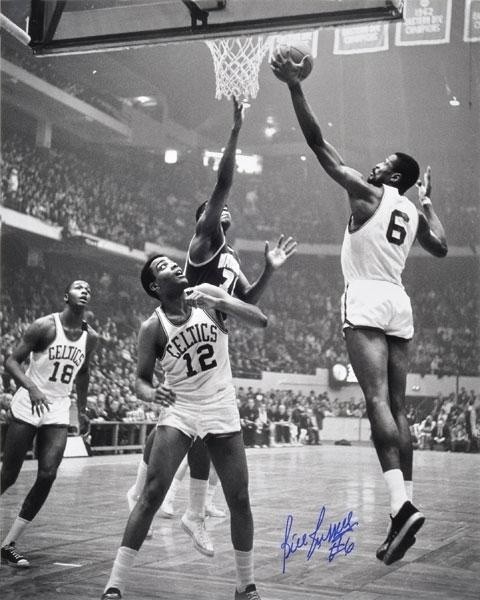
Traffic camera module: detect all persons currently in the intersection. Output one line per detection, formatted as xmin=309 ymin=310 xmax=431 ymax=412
xmin=102 ymin=254 xmax=269 ymax=596
xmin=128 ymin=418 xmax=189 ymax=540
xmin=176 ymin=93 xmax=299 ymax=557
xmin=0 ymin=278 xmax=96 ymax=568
xmin=269 ymin=42 xmax=447 ymax=565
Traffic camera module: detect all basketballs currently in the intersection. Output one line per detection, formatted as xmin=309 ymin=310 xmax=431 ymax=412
xmin=273 ymin=37 xmax=314 ymax=82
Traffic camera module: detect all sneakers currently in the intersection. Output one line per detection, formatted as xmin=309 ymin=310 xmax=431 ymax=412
xmin=181 ymin=509 xmax=215 ymax=557
xmin=235 ymin=584 xmax=260 ymax=600
xmin=127 ymin=484 xmax=152 ymax=540
xmin=101 ymin=588 xmax=122 ymax=600
xmin=383 ymin=501 xmax=425 ymax=565
xmin=376 ymin=534 xmax=416 ymax=561
xmin=0 ymin=541 xmax=29 ymax=568
xmin=414 ymin=442 xmax=478 ymax=455
xmin=160 ymin=500 xmax=175 ymax=518
xmin=204 ymin=503 xmax=227 ymax=518
xmin=247 ymin=440 xmax=322 ymax=448
